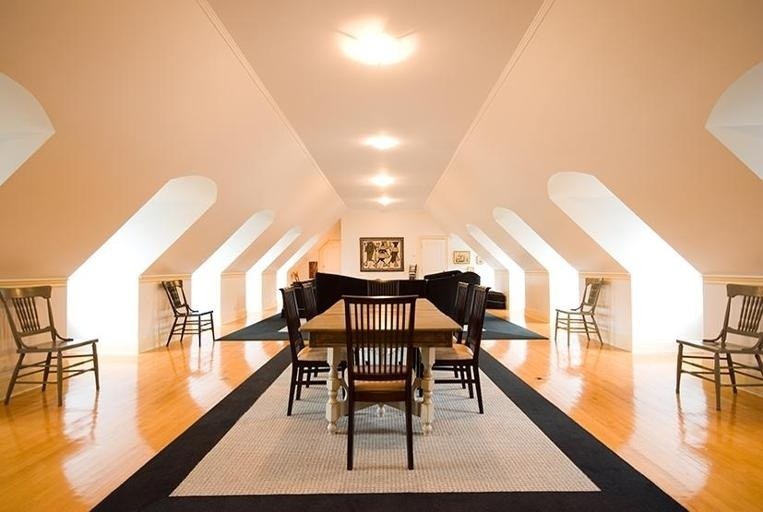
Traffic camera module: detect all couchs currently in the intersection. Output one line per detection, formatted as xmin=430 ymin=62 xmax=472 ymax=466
xmin=280 ymin=270 xmax=507 ymax=318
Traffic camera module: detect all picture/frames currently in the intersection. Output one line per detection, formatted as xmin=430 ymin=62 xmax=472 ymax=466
xmin=360 ymin=236 xmax=404 ymax=272
xmin=453 ymin=250 xmax=470 ymax=263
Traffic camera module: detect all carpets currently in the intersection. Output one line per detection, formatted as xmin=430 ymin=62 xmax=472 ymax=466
xmin=214 ymin=310 xmax=551 ymax=340
xmin=90 ymin=346 xmax=691 ymax=512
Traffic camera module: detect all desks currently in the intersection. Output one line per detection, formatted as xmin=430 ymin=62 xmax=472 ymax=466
xmin=298 ymin=296 xmax=464 ymax=437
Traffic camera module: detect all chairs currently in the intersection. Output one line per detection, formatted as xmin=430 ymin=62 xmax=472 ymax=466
xmin=0 ymin=284 xmax=100 ymax=406
xmin=162 ymin=280 xmax=215 ymax=347
xmin=433 ymin=282 xmax=470 ymax=393
xmin=676 ymin=283 xmax=763 ymax=411
xmin=300 ymin=282 xmax=330 ymax=387
xmin=554 ymin=277 xmax=604 ymax=345
xmin=279 ymin=287 xmax=347 ymax=416
xmin=342 ymin=294 xmax=419 ymax=471
xmin=418 ymin=285 xmax=491 ymax=413
